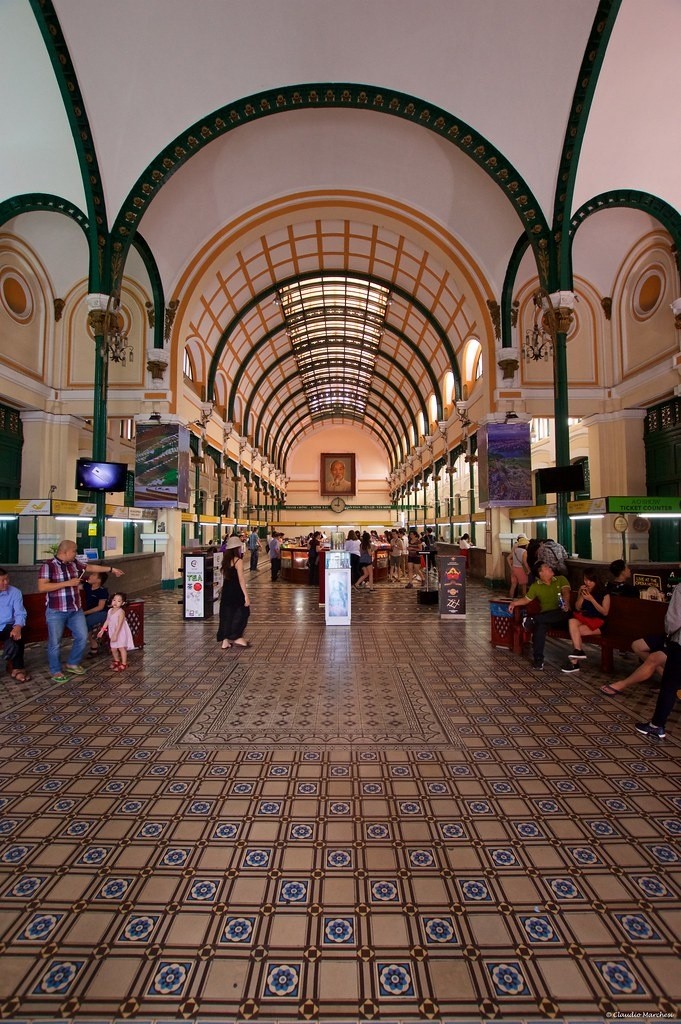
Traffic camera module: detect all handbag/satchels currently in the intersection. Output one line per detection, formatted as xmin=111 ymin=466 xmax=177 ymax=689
xmin=522 ymin=564 xmax=531 ymax=575
xmin=557 ymin=563 xmax=569 ymax=577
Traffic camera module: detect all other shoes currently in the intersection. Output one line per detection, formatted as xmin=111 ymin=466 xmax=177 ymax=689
xmin=353 ymin=585 xmax=360 ymax=592
xmin=405 ymin=583 xmax=413 ymax=588
xmin=421 ymin=580 xmax=426 ymax=586
xmin=370 ymin=589 xmax=378 ymax=593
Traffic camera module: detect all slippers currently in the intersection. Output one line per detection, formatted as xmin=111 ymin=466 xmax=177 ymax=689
xmin=51 ymin=675 xmax=69 ymax=683
xmin=598 ymin=684 xmax=624 ymax=696
xmin=236 ymin=641 xmax=252 ymax=647
xmin=221 ymin=643 xmax=233 ymax=649
xmin=64 ymin=665 xmax=85 ymax=674
xmin=11 ymin=671 xmax=31 ymax=682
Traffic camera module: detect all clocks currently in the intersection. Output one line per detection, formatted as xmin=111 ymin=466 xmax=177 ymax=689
xmin=331 ymin=497 xmax=345 ymax=513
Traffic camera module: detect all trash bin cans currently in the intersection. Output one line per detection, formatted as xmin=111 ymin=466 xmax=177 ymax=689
xmin=488 ymin=597 xmax=516 ymax=649
xmin=122 ymin=599 xmax=146 ymax=649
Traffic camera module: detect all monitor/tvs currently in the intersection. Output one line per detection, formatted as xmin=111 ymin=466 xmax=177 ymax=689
xmin=75 ymin=459 xmax=128 ymax=492
xmin=538 ymin=465 xmax=585 ymax=494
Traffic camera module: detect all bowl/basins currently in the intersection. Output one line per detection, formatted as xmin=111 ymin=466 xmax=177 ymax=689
xmin=572 ymin=554 xmax=578 ymax=559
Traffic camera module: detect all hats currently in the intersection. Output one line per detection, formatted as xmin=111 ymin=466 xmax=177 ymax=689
xmin=517 ymin=538 xmax=530 ymax=546
xmin=517 ymin=534 xmax=530 ymax=541
xmin=226 ymin=536 xmax=242 ymax=549
xmin=426 ymin=528 xmax=432 ymax=533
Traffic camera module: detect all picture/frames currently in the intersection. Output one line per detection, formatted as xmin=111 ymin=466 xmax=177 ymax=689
xmin=320 ymin=452 xmax=356 ymax=496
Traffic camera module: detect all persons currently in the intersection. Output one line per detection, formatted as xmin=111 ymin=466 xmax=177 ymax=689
xmin=381 ymin=528 xmax=409 ymax=582
xmin=250 ymin=528 xmax=262 ymax=572
xmin=405 ymin=530 xmax=425 ymax=588
xmin=508 ymin=561 xmax=572 ymax=670
xmin=562 ymin=559 xmax=681 ymax=738
xmin=353 ymin=533 xmax=377 ymax=593
xmin=268 ymin=531 xmax=284 ymax=582
xmin=346 ymin=531 xmax=362 ymax=587
xmin=97 ymin=592 xmax=135 ymax=672
xmin=220 ymin=533 xmax=246 ymax=560
xmin=460 ymin=533 xmax=471 ymax=578
xmin=355 ymin=531 xmax=360 ymax=539
xmin=506 ymin=534 xmax=568 ymax=596
xmin=37 ymin=540 xmax=125 ymax=682
xmin=307 ymin=531 xmax=327 ymax=585
xmin=0 ymin=568 xmax=32 ymax=683
xmin=421 ymin=528 xmax=437 ymax=574
xmin=79 ymin=573 xmax=109 ymax=658
xmin=371 ymin=531 xmax=378 ymax=539
xmin=217 ymin=537 xmax=251 ymax=649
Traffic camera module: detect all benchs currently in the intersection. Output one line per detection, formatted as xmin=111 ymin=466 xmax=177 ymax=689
xmin=524 ymin=588 xmax=669 ymax=676
xmin=0 ymin=587 xmax=109 ymax=675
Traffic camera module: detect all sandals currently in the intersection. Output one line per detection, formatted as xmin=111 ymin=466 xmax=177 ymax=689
xmin=115 ymin=664 xmax=128 ymax=671
xmin=87 ymin=644 xmax=99 ymax=659
xmin=109 ymin=661 xmax=121 ymax=669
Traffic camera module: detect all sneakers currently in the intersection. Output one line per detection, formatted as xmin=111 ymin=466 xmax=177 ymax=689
xmin=561 ymin=662 xmax=580 ymax=673
xmin=533 ymin=656 xmax=544 ymax=670
xmin=522 ymin=617 xmax=534 ymax=628
xmin=634 ymin=722 xmax=666 ymax=738
xmin=568 ymin=649 xmax=587 ymax=659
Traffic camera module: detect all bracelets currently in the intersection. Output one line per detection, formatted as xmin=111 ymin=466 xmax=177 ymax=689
xmin=101 ymin=628 xmax=107 ymax=631
xmin=110 ymin=567 xmax=112 ymax=572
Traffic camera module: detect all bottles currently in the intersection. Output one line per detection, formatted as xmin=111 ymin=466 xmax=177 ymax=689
xmin=558 ymin=593 xmax=564 ymax=608
